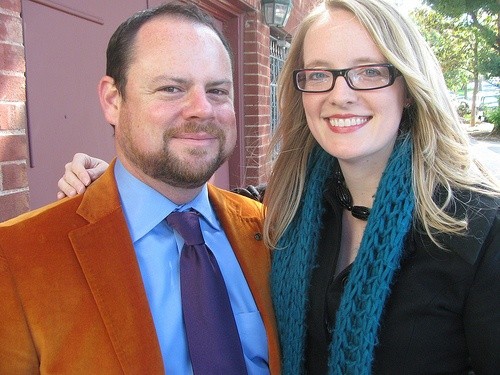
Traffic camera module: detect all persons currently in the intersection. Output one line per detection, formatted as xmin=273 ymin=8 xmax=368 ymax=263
xmin=56 ymin=0 xmax=499 ymax=374
xmin=1 ymin=1 xmax=286 ymax=375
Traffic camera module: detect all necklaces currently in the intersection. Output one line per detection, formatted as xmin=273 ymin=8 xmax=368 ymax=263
xmin=332 ymin=157 xmax=371 ymax=220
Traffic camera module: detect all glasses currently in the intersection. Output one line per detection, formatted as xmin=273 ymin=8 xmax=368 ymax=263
xmin=293 ymin=63 xmax=402 ymax=93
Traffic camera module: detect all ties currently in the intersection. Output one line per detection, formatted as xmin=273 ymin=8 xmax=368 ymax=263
xmin=167 ymin=212 xmax=248 ymax=375
